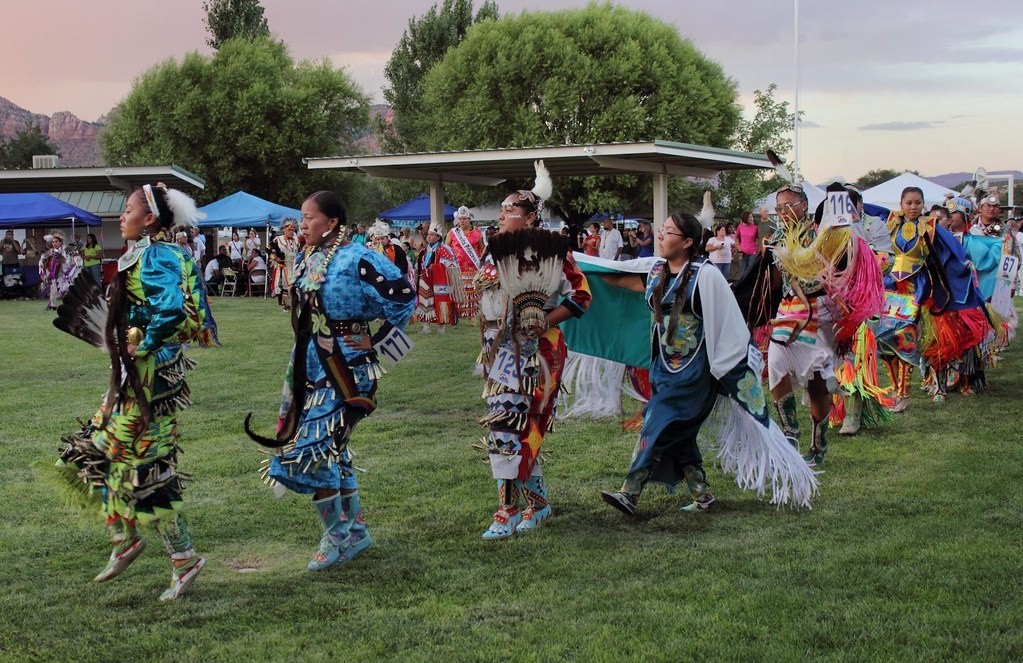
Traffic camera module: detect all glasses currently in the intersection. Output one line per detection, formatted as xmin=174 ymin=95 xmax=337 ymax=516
xmin=659 ymin=228 xmax=691 ymax=237
xmin=775 ymin=201 xmax=801 ymax=212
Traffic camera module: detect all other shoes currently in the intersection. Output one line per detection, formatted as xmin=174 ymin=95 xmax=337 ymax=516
xmin=92 ymin=538 xmax=146 ymax=582
xmin=161 ymin=552 xmax=206 ymax=602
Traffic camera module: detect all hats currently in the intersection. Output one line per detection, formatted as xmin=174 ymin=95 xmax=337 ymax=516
xmin=48 ymin=229 xmax=66 ymax=241
xmin=176 ymin=231 xmax=187 ymax=238
xmin=457 ymin=206 xmax=474 ymax=219
xmin=946 ymin=198 xmax=973 ymax=217
xmin=429 ymin=222 xmax=442 ymax=236
xmin=279 ymin=217 xmax=299 ymax=231
xmin=373 ymin=218 xmax=391 ymax=237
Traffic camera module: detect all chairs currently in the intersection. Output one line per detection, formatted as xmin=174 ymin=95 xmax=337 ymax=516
xmin=206 ymin=268 xmax=266 ymax=299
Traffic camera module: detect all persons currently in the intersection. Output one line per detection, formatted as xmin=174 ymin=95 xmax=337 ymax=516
xmin=552 ymin=219 xmax=652 ymax=261
xmin=766 ymin=182 xmax=895 ymax=467
xmin=868 ymin=185 xmax=1023 ymax=410
xmin=243 ymin=191 xmax=417 ymax=569
xmin=0 ymin=230 xmax=102 ymax=311
xmin=172 ymin=217 xmax=306 ymax=312
xmin=703 ymin=205 xmax=776 ymax=279
xmin=345 ymin=207 xmax=500 ymax=337
xmin=474 ymin=191 xmax=592 ymax=540
xmin=595 ymin=212 xmax=768 ymax=514
xmin=52 ymin=183 xmax=207 ymax=601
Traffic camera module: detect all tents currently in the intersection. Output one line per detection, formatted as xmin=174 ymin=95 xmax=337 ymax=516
xmin=379 ymin=192 xmax=503 ymax=221
xmin=752 ymin=171 xmax=960 ymax=220
xmin=0 ymin=192 xmax=103 ymax=243
xmin=193 ymin=189 xmax=304 ymax=227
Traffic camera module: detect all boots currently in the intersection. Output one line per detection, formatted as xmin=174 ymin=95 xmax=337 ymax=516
xmin=514 ymin=464 xmax=552 ymax=532
xmin=483 ymin=455 xmax=523 ymax=541
xmin=601 ymin=456 xmax=661 ymax=516
xmin=877 ymin=349 xmax=1001 ymax=413
xmin=804 ymin=412 xmax=830 ymax=467
xmin=840 ymin=386 xmax=864 ymax=435
xmin=774 ymin=391 xmax=800 ymax=446
xmin=308 ymin=489 xmax=351 ymax=571
xmin=679 ymin=462 xmax=715 ymax=512
xmin=338 ymin=489 xmax=371 ymax=563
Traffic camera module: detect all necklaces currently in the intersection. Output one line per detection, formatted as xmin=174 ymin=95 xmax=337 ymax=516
xmin=292 ymin=232 xmax=343 ymax=291
